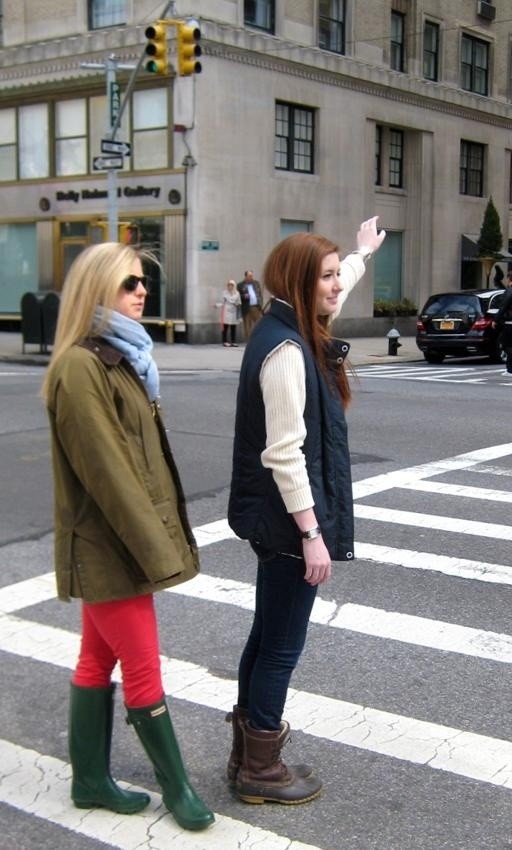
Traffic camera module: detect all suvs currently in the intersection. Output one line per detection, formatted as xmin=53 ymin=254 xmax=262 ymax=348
xmin=416 ymin=287 xmax=511 ymax=366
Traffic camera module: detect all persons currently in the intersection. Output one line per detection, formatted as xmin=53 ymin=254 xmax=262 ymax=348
xmin=230 ymin=214 xmax=389 ymax=805
xmin=239 ymin=270 xmax=265 ymax=341
xmin=492 ymin=272 xmax=511 ymax=377
xmin=215 ymin=280 xmax=242 ymax=347
xmin=40 ymin=241 xmax=216 ymax=830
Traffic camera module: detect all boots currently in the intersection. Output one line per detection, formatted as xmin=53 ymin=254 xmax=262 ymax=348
xmin=225 ymin=703 xmax=327 ymax=806
xmin=124 ymin=690 xmax=219 ymax=832
xmin=68 ymin=679 xmax=151 ymax=815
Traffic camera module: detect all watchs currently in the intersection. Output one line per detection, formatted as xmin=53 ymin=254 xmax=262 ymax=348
xmin=300 ymin=526 xmax=320 ymax=540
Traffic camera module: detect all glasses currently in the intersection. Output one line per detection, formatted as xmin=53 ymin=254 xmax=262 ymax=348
xmin=122 ymin=275 xmax=149 ymax=294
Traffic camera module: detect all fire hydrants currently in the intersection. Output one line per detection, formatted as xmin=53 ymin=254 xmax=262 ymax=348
xmin=387 ymin=328 xmax=402 ymax=357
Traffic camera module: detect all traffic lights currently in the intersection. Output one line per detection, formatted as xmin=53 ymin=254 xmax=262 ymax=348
xmin=145 ymin=24 xmax=169 ymax=78
xmin=177 ymin=25 xmax=202 ymax=77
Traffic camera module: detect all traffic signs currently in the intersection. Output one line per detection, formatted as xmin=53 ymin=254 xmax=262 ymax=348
xmin=92 ymin=155 xmax=123 ymax=171
xmin=100 ymin=139 xmax=131 ymax=156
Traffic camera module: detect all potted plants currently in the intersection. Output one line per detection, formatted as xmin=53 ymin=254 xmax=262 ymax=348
xmin=371 ymin=295 xmax=419 ymax=317
